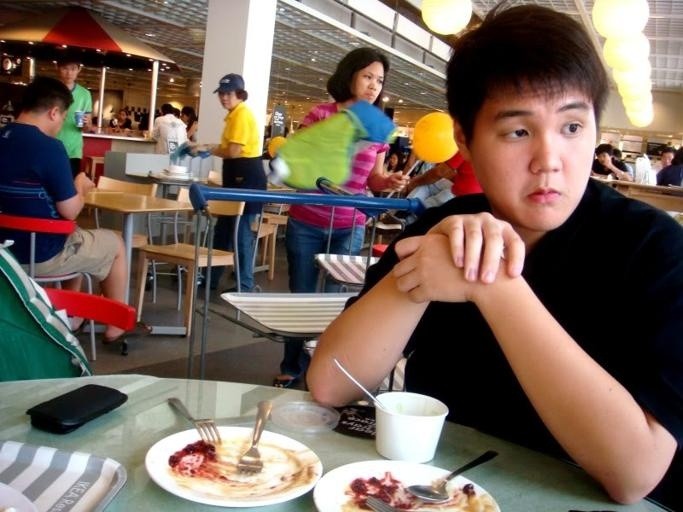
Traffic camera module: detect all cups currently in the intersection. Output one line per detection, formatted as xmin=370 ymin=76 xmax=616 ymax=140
xmin=74 ymin=111 xmax=85 ymax=128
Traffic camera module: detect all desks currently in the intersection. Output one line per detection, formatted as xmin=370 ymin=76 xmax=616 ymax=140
xmin=83 ymin=192 xmax=194 ymax=355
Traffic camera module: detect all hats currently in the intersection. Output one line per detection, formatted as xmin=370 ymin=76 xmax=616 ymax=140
xmin=213 ymin=73 xmax=244 ymax=94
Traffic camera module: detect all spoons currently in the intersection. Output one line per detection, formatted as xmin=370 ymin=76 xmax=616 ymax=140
xmin=406 ymin=450 xmax=499 ymax=502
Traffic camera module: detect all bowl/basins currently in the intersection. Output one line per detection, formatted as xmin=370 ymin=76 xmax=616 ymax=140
xmin=373 ymin=391 xmax=449 ymax=463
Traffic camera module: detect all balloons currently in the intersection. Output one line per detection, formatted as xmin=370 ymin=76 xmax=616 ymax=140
xmin=414 ymin=111 xmax=458 ymax=162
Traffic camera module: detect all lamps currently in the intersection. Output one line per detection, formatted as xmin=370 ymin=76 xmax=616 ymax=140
xmin=601 ymin=34 xmax=650 ymax=70
xmin=613 ymin=70 xmax=657 ymax=130
xmin=423 ymin=1 xmax=472 ymax=35
xmin=592 ymin=0 xmax=649 ymax=39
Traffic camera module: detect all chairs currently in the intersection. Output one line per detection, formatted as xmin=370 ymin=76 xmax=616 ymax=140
xmin=0 ymin=215 xmax=100 ymax=361
xmin=91 ymin=175 xmax=158 ymax=296
xmin=134 ymin=200 xmax=245 ymax=338
xmin=253 ymin=205 xmax=279 ymax=284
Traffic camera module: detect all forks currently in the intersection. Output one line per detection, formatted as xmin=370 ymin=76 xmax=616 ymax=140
xmin=168 ymin=397 xmax=223 ymax=445
xmin=236 ymin=400 xmax=274 ymax=477
xmin=364 ymin=495 xmax=396 ymax=512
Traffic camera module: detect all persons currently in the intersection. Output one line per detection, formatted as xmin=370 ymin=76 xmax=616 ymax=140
xmin=109 ymin=109 xmax=132 ymax=129
xmin=0 ymin=77 xmax=153 ymax=350
xmin=153 ymin=103 xmax=187 ymax=154
xmin=189 ymin=74 xmax=269 ymax=293
xmin=380 ymin=152 xmax=404 ymax=198
xmin=654 ymin=147 xmax=676 ymax=175
xmin=55 ymin=53 xmax=92 ymax=181
xmin=592 ymin=144 xmax=631 ymax=182
xmin=657 ymin=145 xmax=683 ymax=187
xmin=124 ymin=106 xmax=161 ymax=121
xmin=91 ymin=116 xmax=103 ymax=134
xmin=306 ymin=4 xmax=682 ymax=512
xmin=272 ymin=50 xmax=410 ymax=389
xmin=269 ymin=136 xmax=286 ymax=157
xmin=612 ymin=148 xmax=633 ymax=178
xmin=370 ymin=149 xmax=481 ymax=234
xmin=173 ymin=107 xmax=188 ymax=129
xmin=181 ymin=106 xmax=197 ymax=141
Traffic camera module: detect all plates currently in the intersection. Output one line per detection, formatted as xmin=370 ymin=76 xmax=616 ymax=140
xmin=148 ymin=169 xmax=199 ymax=185
xmin=312 ymin=253 xmax=380 ymax=287
xmin=142 ymin=425 xmax=323 ymax=507
xmin=312 ymin=458 xmax=500 ymax=512
xmin=222 ymin=291 xmax=359 ymax=337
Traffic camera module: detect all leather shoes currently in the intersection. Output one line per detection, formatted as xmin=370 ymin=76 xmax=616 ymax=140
xmin=273 ymin=377 xmax=298 ymax=389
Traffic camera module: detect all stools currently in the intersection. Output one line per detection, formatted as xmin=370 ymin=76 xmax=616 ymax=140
xmin=85 ymin=156 xmax=104 ymax=181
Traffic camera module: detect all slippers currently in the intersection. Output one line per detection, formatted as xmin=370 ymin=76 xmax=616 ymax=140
xmin=73 ymin=319 xmax=88 ymax=335
xmin=102 ymin=322 xmax=153 ymax=345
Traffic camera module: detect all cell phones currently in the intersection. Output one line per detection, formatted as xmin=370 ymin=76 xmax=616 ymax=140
xmin=26 ymin=382 xmax=127 ymax=435
